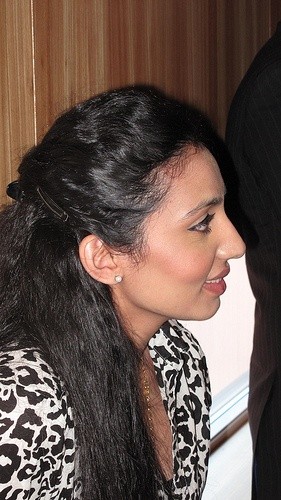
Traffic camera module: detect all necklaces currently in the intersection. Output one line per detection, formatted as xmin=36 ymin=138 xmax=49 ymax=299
xmin=140 ymin=362 xmax=156 ymax=440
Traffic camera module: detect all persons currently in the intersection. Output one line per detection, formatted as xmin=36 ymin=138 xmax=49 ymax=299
xmin=225 ymin=28 xmax=281 ymax=500
xmin=0 ymin=83 xmax=246 ymax=500
